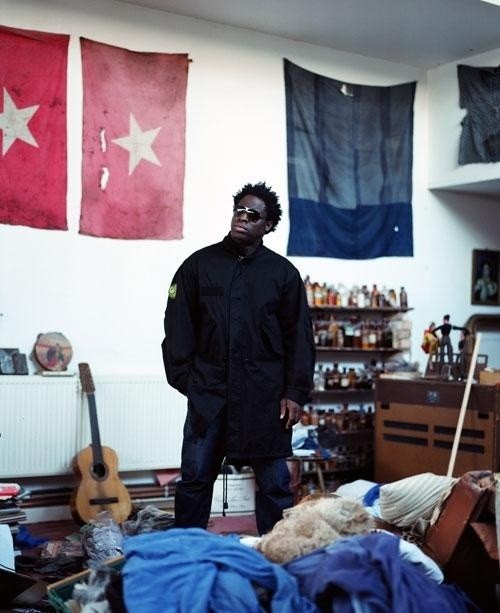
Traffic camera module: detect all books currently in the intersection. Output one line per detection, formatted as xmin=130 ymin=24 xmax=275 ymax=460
xmin=0 ymin=483 xmax=27 ymax=559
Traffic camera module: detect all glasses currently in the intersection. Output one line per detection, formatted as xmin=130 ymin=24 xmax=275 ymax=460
xmin=233 ymin=203 xmax=269 ymax=224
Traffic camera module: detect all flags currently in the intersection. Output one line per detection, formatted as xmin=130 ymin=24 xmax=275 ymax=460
xmin=1 ymin=26 xmax=68 ymax=230
xmin=78 ymin=38 xmax=189 ymax=240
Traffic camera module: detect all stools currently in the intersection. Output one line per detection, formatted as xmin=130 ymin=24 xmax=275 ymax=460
xmin=289 ymin=453 xmax=328 ymax=502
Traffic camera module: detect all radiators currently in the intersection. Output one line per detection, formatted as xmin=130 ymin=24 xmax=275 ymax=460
xmin=0 ymin=371 xmax=189 ymax=479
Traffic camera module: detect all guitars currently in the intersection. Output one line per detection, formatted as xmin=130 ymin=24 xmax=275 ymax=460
xmin=70 ymin=364 xmax=132 ymax=526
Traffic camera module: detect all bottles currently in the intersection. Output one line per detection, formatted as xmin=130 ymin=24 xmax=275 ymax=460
xmin=302 ymin=274 xmax=413 ymax=492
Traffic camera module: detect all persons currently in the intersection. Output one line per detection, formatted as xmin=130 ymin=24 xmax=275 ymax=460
xmin=473 ymin=263 xmax=498 ymax=300
xmin=422 ymin=315 xmax=476 ymax=380
xmin=46 ymin=342 xmax=63 ymax=362
xmin=162 ymin=182 xmax=316 ymax=538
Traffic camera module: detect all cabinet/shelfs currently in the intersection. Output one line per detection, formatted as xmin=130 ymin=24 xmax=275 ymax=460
xmin=303 ymin=300 xmax=415 ymax=478
xmin=371 ymin=367 xmax=500 ymax=484
